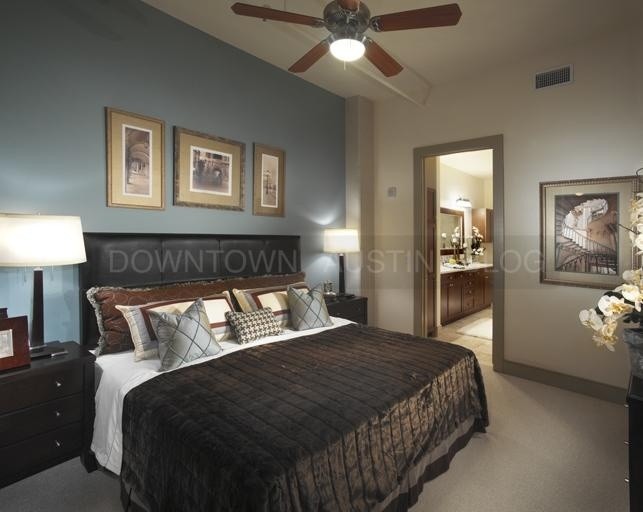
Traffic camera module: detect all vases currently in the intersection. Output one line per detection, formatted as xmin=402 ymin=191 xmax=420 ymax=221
xmin=622 ymin=325 xmax=643 ymax=383
xmin=471 ymin=253 xmax=480 ymax=265
xmin=451 ymin=244 xmax=460 ymax=263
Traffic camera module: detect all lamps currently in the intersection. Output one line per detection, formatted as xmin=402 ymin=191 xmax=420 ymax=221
xmin=453 ymin=196 xmax=472 ymax=209
xmin=0 ymin=210 xmax=88 ymax=362
xmin=321 ymin=228 xmax=362 ymax=302
xmin=320 ymin=10 xmax=371 ymax=65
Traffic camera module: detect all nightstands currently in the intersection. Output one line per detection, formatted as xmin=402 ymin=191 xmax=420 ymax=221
xmin=320 ymin=290 xmax=368 ymax=325
xmin=0 ymin=340 xmax=101 ymax=492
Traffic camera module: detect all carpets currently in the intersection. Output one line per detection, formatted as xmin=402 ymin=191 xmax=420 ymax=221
xmin=454 ymin=315 xmax=493 ymax=342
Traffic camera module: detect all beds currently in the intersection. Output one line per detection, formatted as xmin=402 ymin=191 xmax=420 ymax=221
xmin=78 ymin=229 xmax=489 ymax=510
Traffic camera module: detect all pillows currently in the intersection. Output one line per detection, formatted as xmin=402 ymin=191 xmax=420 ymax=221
xmin=146 ymin=297 xmax=225 ymax=373
xmin=114 ymin=289 xmax=240 ymax=361
xmin=224 ymin=304 xmax=284 ymax=347
xmin=82 ymin=269 xmax=307 ymax=356
xmin=231 ymin=278 xmax=314 ymax=332
xmin=286 ymin=283 xmax=334 ymax=332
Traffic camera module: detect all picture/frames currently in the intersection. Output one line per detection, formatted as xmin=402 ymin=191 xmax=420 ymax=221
xmin=105 ymin=107 xmax=165 ymax=211
xmin=253 ymin=142 xmax=286 ymax=218
xmin=173 ymin=124 xmax=246 ymax=212
xmin=0 ymin=314 xmax=32 ymax=371
xmin=538 ymin=175 xmax=643 ymax=292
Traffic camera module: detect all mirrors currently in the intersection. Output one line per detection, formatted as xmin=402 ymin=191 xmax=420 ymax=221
xmin=438 ymin=206 xmax=465 ymax=255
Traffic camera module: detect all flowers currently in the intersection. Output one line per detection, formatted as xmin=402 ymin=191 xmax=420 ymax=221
xmin=441 ymin=224 xmax=487 ymax=256
xmin=573 ymin=189 xmax=643 ymax=353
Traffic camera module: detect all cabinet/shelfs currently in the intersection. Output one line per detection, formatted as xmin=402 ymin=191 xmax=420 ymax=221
xmin=621 ymin=367 xmax=642 ymax=511
xmin=439 ymin=272 xmax=461 ymax=326
xmin=471 ymin=207 xmax=494 ymax=244
xmin=482 ymin=266 xmax=493 ymax=311
xmin=461 ymin=266 xmax=483 ymax=317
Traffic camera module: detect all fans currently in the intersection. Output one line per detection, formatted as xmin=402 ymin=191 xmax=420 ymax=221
xmin=228 ymin=0 xmax=463 ymax=77
